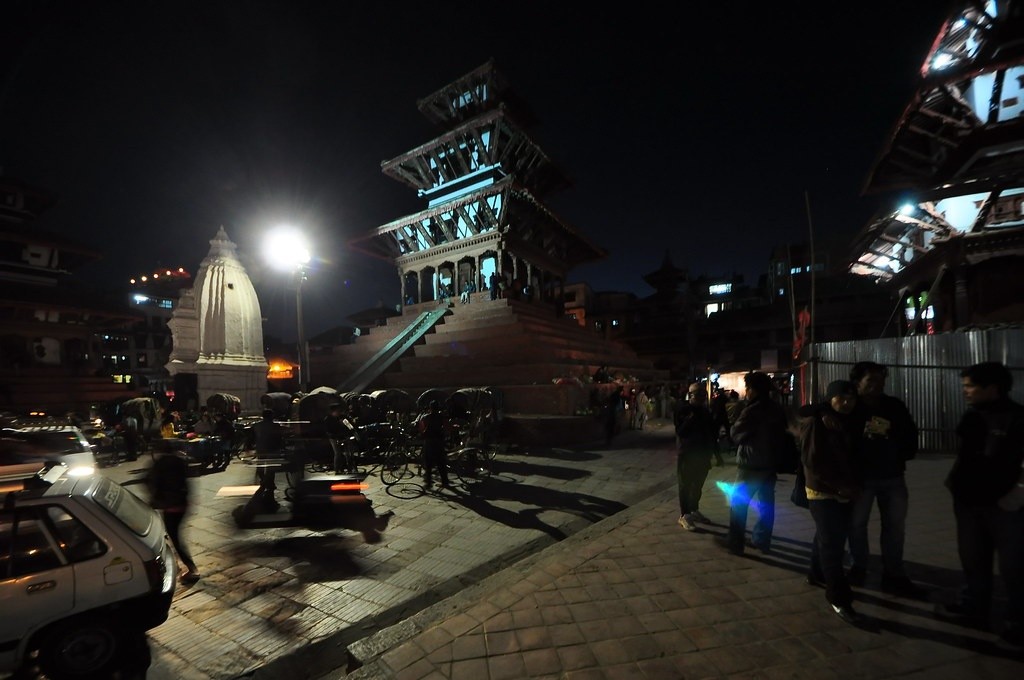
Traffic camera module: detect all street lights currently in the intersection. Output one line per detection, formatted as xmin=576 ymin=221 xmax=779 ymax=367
xmin=267 ymin=225 xmax=308 ymax=394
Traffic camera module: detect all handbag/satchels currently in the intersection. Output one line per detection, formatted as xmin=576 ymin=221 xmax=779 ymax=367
xmin=791 ymin=471 xmax=810 ymax=510
xmin=776 ymin=432 xmax=800 ymax=473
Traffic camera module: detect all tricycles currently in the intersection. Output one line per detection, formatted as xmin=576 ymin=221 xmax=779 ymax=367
xmin=117 ymin=384 xmax=498 ymax=486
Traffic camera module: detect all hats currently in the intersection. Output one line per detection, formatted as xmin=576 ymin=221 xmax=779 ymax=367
xmin=685 ymin=381 xmax=707 ymax=397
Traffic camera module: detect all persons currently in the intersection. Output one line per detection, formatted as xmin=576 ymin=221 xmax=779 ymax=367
xmin=795 ymin=362 xmax=921 ymax=622
xmin=673 ymin=372 xmax=782 ymax=555
xmin=417 ymin=400 xmax=449 ymax=488
xmin=592 ymin=365 xmax=649 ymax=449
xmin=253 ymin=410 xmax=281 ymax=496
xmin=396 ymin=304 xmax=400 ymax=316
xmin=322 ymin=408 xmax=357 ymax=474
xmin=350 ymin=325 xmax=360 ymax=344
xmin=160 ymin=411 xmax=234 ymax=439
xmin=439 ymin=280 xmax=475 ymax=304
xmin=490 ymin=272 xmax=532 ymax=304
xmin=932 ymin=362 xmax=1024 ymax=650
xmin=144 ymin=440 xmax=200 ymax=580
xmin=121 ymin=417 xmax=137 ymax=462
xmin=405 ymin=293 xmax=414 ymax=304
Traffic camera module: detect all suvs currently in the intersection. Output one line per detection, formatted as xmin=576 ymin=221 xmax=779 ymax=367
xmin=0 ymin=461 xmax=176 ymax=680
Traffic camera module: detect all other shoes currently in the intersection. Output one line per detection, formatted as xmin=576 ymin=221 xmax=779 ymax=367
xmin=693 ymin=509 xmax=712 ymax=526
xmin=937 ymin=588 xmax=992 ymax=616
xmin=712 ymin=531 xmax=741 ymax=553
xmin=677 ymin=514 xmax=697 ymax=530
xmin=999 ymin=628 xmax=1023 ymax=648
xmin=808 ymin=574 xmax=828 ymax=589
xmin=889 ymin=575 xmax=925 ymax=600
xmin=832 ymin=603 xmax=864 ymax=622
xmin=177 ymin=572 xmax=200 ymax=580
xmin=747 ymin=532 xmax=770 ymax=554
xmin=848 ymin=557 xmax=870 ymax=584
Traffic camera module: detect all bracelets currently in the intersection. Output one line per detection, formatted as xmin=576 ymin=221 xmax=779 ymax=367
xmin=1017 ymin=483 xmax=1024 ymax=488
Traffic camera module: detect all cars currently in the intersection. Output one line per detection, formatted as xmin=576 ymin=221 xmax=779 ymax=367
xmin=6 ymin=426 xmax=100 ymax=477
